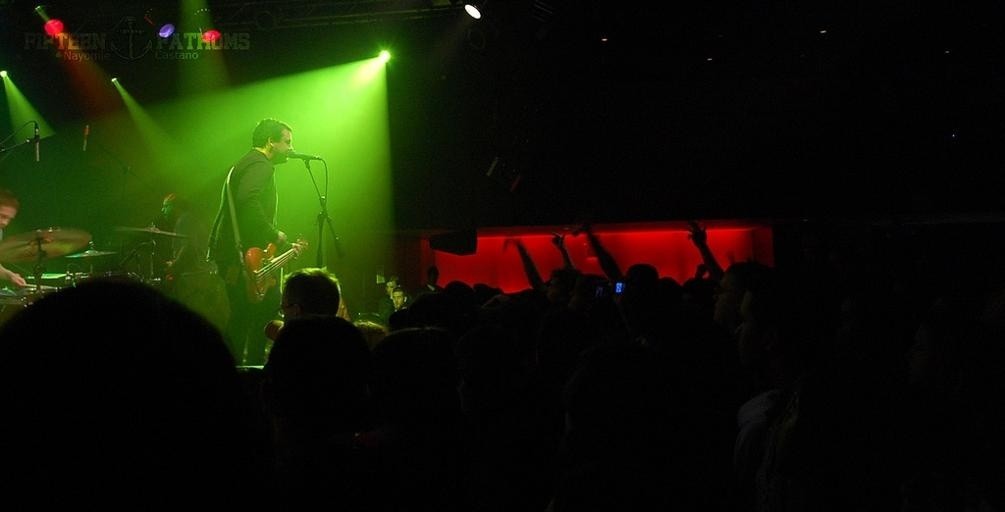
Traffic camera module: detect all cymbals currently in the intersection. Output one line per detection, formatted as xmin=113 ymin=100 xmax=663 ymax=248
xmin=63 ymin=250 xmax=116 ymax=260
xmin=112 ymin=225 xmax=187 ymax=240
xmin=0 ymin=226 xmax=92 ymax=265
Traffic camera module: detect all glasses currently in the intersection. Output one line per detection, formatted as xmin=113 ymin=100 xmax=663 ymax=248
xmin=270 ymin=304 xmax=293 ymax=318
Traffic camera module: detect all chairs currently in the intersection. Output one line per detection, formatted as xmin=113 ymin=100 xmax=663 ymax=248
xmin=258 ymin=330 xmax=656 ymax=510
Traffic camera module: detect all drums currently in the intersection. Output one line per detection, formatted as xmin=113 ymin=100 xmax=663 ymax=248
xmin=0 ymin=285 xmax=62 ymax=326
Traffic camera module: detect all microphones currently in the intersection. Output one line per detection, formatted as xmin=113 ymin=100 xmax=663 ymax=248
xmin=35 ymin=123 xmax=40 ymax=162
xmin=288 ymin=152 xmax=321 ymax=160
xmin=82 ymin=124 xmax=90 ymax=151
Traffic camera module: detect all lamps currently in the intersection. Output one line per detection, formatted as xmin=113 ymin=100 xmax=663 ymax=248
xmin=463 ymin=0 xmax=487 ymax=19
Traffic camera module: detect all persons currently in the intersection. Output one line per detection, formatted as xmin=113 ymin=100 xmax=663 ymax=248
xmin=1 ymin=261 xmax=1003 ymax=511
xmin=161 ymin=121 xmax=293 ymax=346
xmin=0 ymin=188 xmax=27 ymax=289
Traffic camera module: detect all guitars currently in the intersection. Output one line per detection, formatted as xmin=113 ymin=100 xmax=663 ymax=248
xmin=245 ymin=234 xmax=308 ymax=304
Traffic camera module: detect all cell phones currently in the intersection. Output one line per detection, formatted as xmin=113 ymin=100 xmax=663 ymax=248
xmin=614 ymin=280 xmax=624 ymax=303
